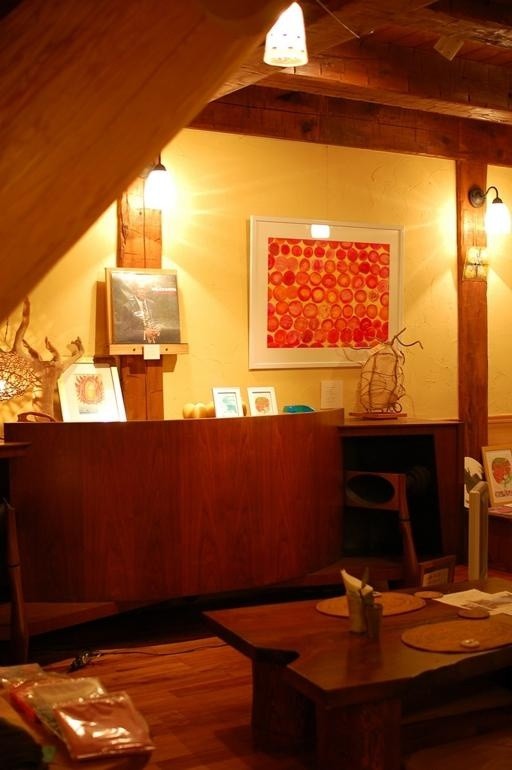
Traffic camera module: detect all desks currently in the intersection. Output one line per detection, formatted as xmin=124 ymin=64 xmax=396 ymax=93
xmin=0 ymin=406 xmax=465 ymax=665
xmin=200 ymin=572 xmax=512 ymax=769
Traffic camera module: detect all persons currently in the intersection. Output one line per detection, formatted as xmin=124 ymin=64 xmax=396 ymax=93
xmin=123 ymin=279 xmax=165 ymax=343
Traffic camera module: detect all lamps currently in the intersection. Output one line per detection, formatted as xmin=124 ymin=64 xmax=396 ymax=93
xmin=141 ymin=148 xmax=181 ymax=213
xmin=261 ymin=3 xmax=311 ymax=69
xmin=469 ymin=182 xmax=511 ymax=248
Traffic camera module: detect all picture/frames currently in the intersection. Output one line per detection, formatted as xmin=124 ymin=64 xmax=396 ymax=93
xmin=211 ymin=385 xmax=245 ymax=421
xmin=102 ymin=265 xmax=191 ymax=357
xmin=56 ymin=356 xmax=128 ymax=424
xmin=246 ymin=384 xmax=282 ymax=419
xmin=247 ymin=215 xmax=402 ymax=373
xmin=481 ymin=444 xmax=512 ymax=512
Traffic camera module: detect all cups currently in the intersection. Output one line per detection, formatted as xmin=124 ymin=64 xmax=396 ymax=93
xmin=365 ymin=603 xmax=383 ymax=643
